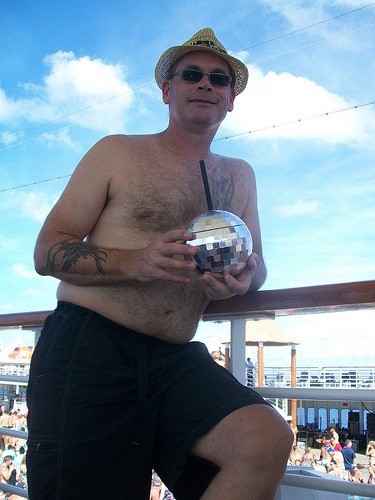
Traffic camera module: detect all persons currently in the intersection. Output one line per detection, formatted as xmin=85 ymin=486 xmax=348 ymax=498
xmin=25 ymin=26 xmax=294 ymax=500
xmin=0 ymin=351 xmax=375 ymax=500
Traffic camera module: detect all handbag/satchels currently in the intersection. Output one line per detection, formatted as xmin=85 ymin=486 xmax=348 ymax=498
xmin=334 ymin=444 xmax=342 ymax=452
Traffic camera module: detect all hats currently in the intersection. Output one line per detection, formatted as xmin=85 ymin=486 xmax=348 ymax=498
xmin=155 ymin=28 xmax=248 ymax=96
xmin=329 ymin=427 xmax=335 ymax=432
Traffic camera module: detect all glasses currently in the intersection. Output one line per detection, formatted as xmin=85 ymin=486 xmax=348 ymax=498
xmin=169 ymin=69 xmax=234 ymax=87
